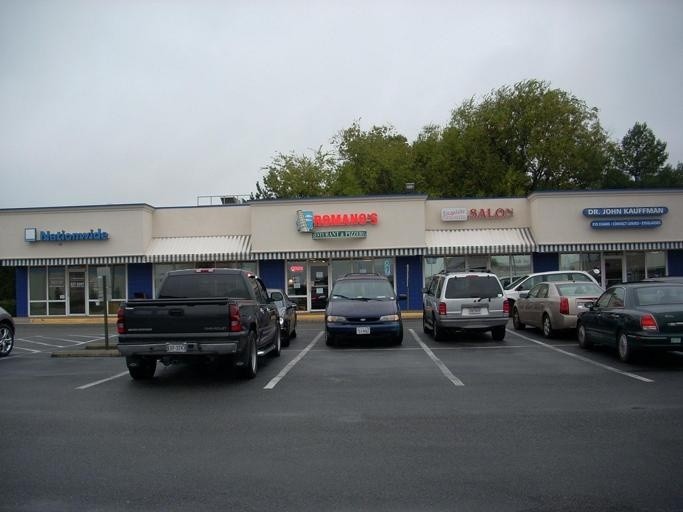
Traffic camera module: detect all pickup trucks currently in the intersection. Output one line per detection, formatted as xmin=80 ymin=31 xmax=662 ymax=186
xmin=116 ymin=268 xmax=283 ymax=380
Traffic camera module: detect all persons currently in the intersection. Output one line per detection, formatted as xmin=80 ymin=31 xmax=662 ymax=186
xmin=55 ymin=285 xmax=62 ymax=305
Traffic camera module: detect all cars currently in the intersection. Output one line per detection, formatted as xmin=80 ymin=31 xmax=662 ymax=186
xmin=512 ymin=281 xmax=606 ymax=339
xmin=0 ymin=306 xmax=15 ymax=357
xmin=576 ymin=276 xmax=683 ymax=363
xmin=264 ymin=288 xmax=296 ymax=347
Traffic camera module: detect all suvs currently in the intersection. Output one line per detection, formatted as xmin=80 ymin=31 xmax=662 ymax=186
xmin=503 ymin=271 xmax=601 ymax=318
xmin=424 ymin=271 xmax=510 ymax=341
xmin=316 ymin=273 xmax=408 ymax=346
